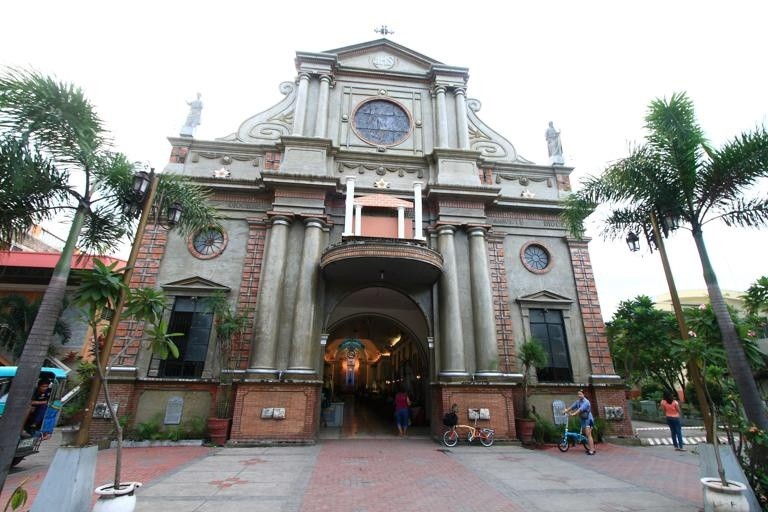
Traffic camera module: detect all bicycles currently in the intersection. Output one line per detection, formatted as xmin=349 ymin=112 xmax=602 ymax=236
xmin=557 ymin=411 xmax=596 ymax=452
xmin=442 ymin=402 xmax=495 ymax=448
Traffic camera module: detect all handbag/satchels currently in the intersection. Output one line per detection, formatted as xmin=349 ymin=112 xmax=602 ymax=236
xmin=580 ymin=411 xmax=588 ymax=419
xmin=443 ymin=411 xmax=459 ymax=428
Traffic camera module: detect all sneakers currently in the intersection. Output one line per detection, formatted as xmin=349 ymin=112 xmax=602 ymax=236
xmin=588 ymin=450 xmax=595 ymax=455
xmin=675 ymin=448 xmax=687 ymax=451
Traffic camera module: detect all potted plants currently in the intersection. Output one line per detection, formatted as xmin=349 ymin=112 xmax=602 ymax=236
xmin=515 ymin=333 xmax=548 ymax=446
xmin=72 ymin=257 xmax=188 ymax=512
xmin=669 ymin=300 xmax=766 ymax=512
xmin=207 ymin=291 xmax=251 ymax=445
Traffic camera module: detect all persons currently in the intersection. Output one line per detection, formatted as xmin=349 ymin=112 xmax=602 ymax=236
xmin=20 ymin=378 xmax=52 ymax=439
xmin=546 ymin=120 xmax=564 ymax=156
xmin=393 ymin=386 xmax=412 ymax=437
xmin=660 ymin=390 xmax=688 ymax=450
xmin=183 ymin=89 xmax=203 ymax=128
xmin=564 ymin=389 xmax=596 ymax=455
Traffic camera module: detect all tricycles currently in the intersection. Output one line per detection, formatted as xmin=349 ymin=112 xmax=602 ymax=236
xmin=0 ymin=365 xmax=68 ymax=467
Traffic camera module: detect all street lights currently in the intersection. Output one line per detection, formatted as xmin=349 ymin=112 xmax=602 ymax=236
xmin=74 ymin=168 xmax=161 ymax=448
xmin=626 ymin=206 xmax=723 ymax=445
